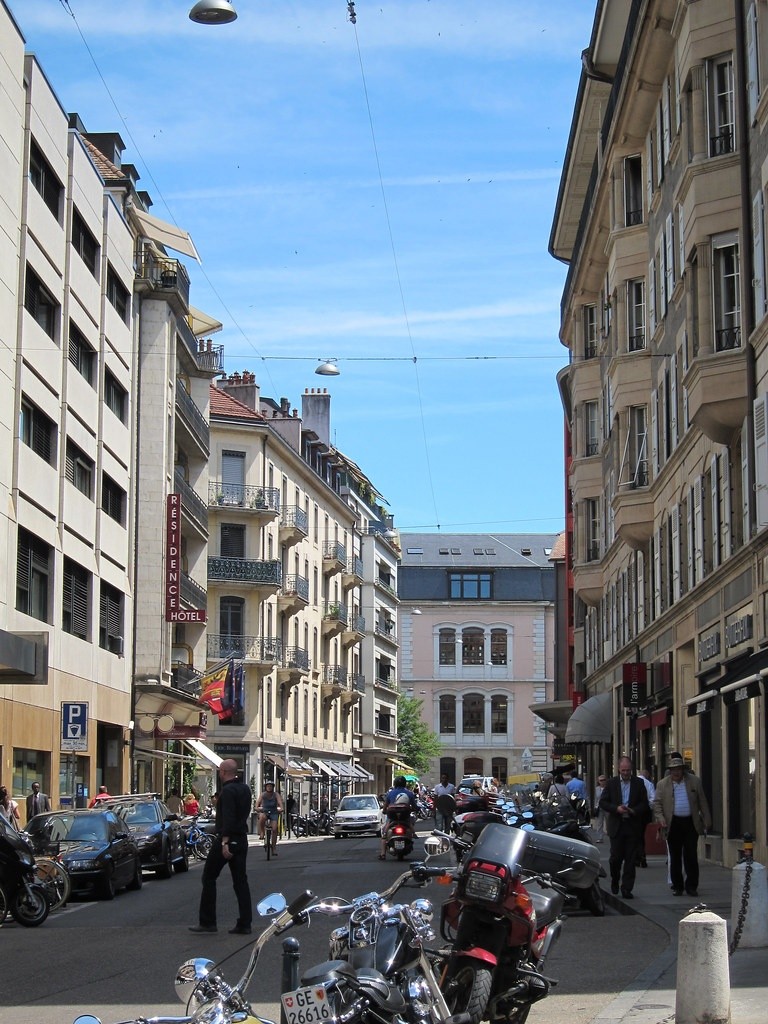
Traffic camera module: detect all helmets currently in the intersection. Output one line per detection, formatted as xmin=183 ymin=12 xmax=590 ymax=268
xmin=393 ymin=775 xmax=407 ymax=788
xmin=394 ymin=792 xmax=410 ymax=807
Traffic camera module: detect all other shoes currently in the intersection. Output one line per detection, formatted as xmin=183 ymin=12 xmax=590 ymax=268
xmin=272 ymin=847 xmax=278 ymax=856
xmin=620 ymin=886 xmax=634 ymax=900
xmin=672 ymin=888 xmax=684 ymax=896
xmin=188 ymin=923 xmax=218 ymax=932
xmin=685 ymin=888 xmax=698 ymax=897
xmin=610 ymin=878 xmax=620 ymax=895
xmin=228 ymin=923 xmax=252 ymax=934
xmin=633 ymin=859 xmax=648 ymax=869
xmin=596 ymin=839 xmax=604 ymax=843
xmin=258 ymin=831 xmax=265 ymax=840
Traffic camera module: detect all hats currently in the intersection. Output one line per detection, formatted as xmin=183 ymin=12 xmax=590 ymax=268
xmin=263 ymin=780 xmax=275 ymax=788
xmin=665 ymin=758 xmax=688 ymax=769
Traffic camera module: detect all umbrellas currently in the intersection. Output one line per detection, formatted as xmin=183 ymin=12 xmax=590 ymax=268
xmin=405 ymin=775 xmax=420 ymax=782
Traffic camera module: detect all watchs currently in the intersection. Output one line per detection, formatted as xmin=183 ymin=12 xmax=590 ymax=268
xmin=222 ymin=842 xmax=228 ymax=846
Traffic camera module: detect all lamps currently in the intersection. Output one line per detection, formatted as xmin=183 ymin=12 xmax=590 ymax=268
xmin=315 ymin=357 xmax=340 ymax=376
xmin=383 ymin=527 xmax=397 ymax=538
xmin=189 ymin=0 xmax=238 ymax=25
xmin=410 ymin=604 xmax=422 ymax=615
xmin=486 ymin=661 xmax=493 ymax=666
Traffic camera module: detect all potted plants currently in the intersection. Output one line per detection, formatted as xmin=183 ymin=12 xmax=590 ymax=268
xmin=217 ymin=494 xmax=223 ymax=504
xmin=255 ymin=497 xmax=262 ymax=508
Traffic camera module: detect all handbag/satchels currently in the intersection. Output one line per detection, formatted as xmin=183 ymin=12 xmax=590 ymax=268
xmin=10 ymin=799 xmax=21 ymax=832
xmin=644 ymin=821 xmax=669 ymax=856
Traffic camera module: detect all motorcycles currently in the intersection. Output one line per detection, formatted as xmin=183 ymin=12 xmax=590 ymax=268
xmin=72 ymin=835 xmax=452 ymax=1023
xmin=428 ymin=791 xmax=609 ymax=1024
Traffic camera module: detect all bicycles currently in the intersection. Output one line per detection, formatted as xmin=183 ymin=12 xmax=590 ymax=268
xmin=252 ymin=806 xmax=284 ymax=860
xmin=181 ymin=811 xmax=219 ymax=861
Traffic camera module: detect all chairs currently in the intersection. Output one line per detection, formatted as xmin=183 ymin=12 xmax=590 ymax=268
xmin=160 ymin=270 xmax=177 ymax=288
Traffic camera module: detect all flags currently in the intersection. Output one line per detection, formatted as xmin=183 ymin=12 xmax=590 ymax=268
xmin=198 ymin=656 xmax=244 ymax=720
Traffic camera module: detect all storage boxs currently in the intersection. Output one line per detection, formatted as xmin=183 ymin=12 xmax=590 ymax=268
xmin=523 ymin=830 xmax=602 ymax=890
xmin=456 ymin=796 xmax=488 ymax=813
xmin=462 ymin=812 xmax=507 ymax=839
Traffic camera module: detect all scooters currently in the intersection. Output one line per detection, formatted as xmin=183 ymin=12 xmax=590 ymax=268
xmin=411 ymin=791 xmax=437 ymax=824
xmin=288 ymin=806 xmax=335 ymax=836
xmin=0 ymin=805 xmax=71 ymax=931
xmin=385 ymin=802 xmax=415 ymax=861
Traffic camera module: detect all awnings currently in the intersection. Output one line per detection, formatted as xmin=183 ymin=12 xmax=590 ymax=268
xmin=387 ymin=758 xmax=413 ymax=769
xmin=185 ymin=304 xmax=224 ymax=339
xmin=136 ymin=739 xmax=223 ymax=771
xmin=266 ymin=754 xmax=374 ymax=782
xmin=338 ymin=450 xmax=391 ymax=507
xmin=564 ymin=694 xmax=612 ymax=744
xmin=130 ymin=204 xmax=201 ymax=283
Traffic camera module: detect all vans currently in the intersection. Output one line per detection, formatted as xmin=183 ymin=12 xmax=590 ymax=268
xmin=456 ymin=774 xmax=494 ymax=795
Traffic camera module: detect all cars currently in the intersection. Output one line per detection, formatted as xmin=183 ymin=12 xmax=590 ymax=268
xmin=333 ymin=793 xmax=388 ymax=839
xmin=88 ymin=792 xmax=190 ymax=880
xmin=26 ymin=808 xmax=143 ymax=900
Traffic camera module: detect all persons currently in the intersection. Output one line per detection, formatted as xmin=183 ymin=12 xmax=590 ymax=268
xmin=26 ymin=782 xmax=51 ymax=823
xmin=0 ymin=785 xmax=21 ymax=827
xmin=434 ymin=775 xmax=455 ymax=834
xmin=594 ymin=752 xmax=711 ymax=900
xmin=288 ymin=795 xmax=296 ymax=825
xmin=414 ymin=783 xmax=433 ymax=809
xmin=538 ymin=772 xmax=589 ymax=825
xmin=256 ymin=782 xmax=283 ymax=855
xmin=166 ymin=787 xmax=199 ymax=816
xmin=189 ymin=759 xmax=252 ymax=933
xmin=88 ymin=785 xmax=112 ymax=809
xmin=473 ymin=778 xmax=503 ymax=796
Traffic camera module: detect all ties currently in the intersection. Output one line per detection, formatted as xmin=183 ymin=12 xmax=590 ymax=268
xmin=33 ymin=795 xmax=39 ymax=816
